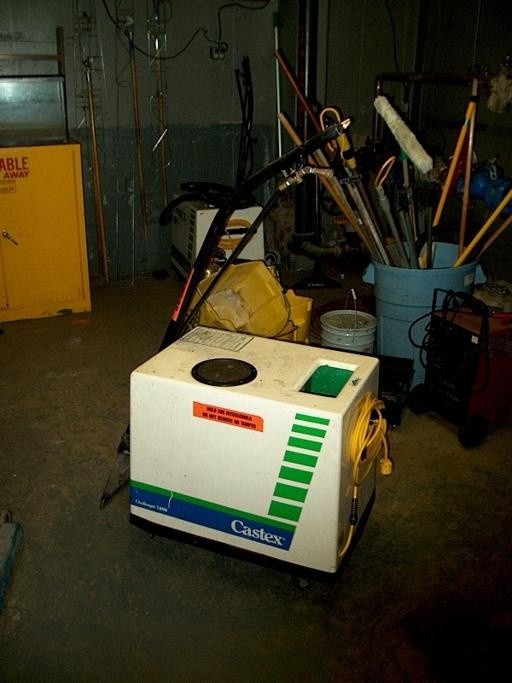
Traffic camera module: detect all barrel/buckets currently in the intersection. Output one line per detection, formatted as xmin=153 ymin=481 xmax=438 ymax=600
xmin=320 ymin=288 xmax=376 ymax=356
xmin=371 ymin=241 xmax=477 ymax=392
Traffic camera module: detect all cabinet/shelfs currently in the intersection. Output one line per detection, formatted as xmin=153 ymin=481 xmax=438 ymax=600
xmin=1 ymin=137 xmax=93 ymax=323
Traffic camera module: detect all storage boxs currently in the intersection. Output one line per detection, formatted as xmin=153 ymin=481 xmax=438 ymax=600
xmin=170 ymin=193 xmax=265 ymax=281
